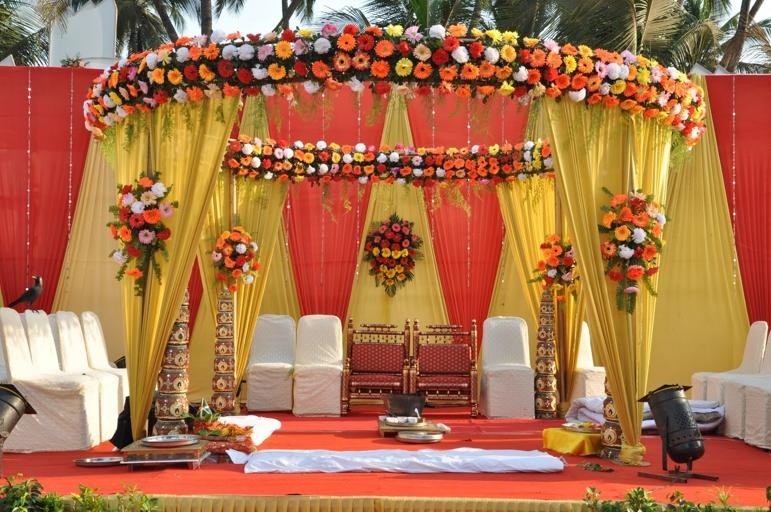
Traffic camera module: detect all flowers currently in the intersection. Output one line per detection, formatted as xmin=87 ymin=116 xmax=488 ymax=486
xmin=366 ymin=218 xmax=420 ymax=292
xmin=601 ymin=189 xmax=667 ymax=295
xmin=82 ymin=23 xmax=706 ymax=145
xmin=222 ymin=134 xmax=554 ymax=185
xmin=212 ymin=226 xmax=261 ymax=295
xmin=535 ymin=234 xmax=579 ymax=302
xmin=108 ymin=172 xmax=178 ymax=297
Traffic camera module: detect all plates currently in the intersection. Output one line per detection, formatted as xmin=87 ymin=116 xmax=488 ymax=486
xmin=143 ymin=436 xmax=201 ymax=447
xmin=75 ymin=458 xmax=123 ymax=466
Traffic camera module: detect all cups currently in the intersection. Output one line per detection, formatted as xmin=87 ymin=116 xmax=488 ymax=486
xmin=379 ymin=416 xmax=418 ymax=423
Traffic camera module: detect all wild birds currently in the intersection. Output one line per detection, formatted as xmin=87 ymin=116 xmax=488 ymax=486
xmin=7 ymin=275 xmax=43 ymax=313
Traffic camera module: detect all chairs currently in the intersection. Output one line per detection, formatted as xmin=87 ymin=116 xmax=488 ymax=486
xmin=571 ymin=322 xmax=606 ymax=403
xmin=341 ymin=318 xmax=410 ymax=414
xmin=293 ymin=314 xmax=343 ymax=417
xmin=479 ymin=315 xmax=535 ymax=421
xmin=410 ymin=318 xmax=479 ymax=418
xmin=690 ymin=320 xmax=771 ymax=451
xmin=0 ymin=303 xmax=131 ymax=455
xmin=246 ymin=314 xmax=296 ymax=411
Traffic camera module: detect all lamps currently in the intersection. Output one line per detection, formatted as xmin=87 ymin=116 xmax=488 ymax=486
xmin=636 ymin=382 xmax=722 ymax=483
xmin=0 ymin=384 xmax=37 ymax=451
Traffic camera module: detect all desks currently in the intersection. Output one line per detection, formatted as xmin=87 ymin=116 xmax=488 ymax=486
xmin=120 ymin=436 xmax=211 ymax=469
xmin=541 ymin=427 xmax=603 ymax=456
